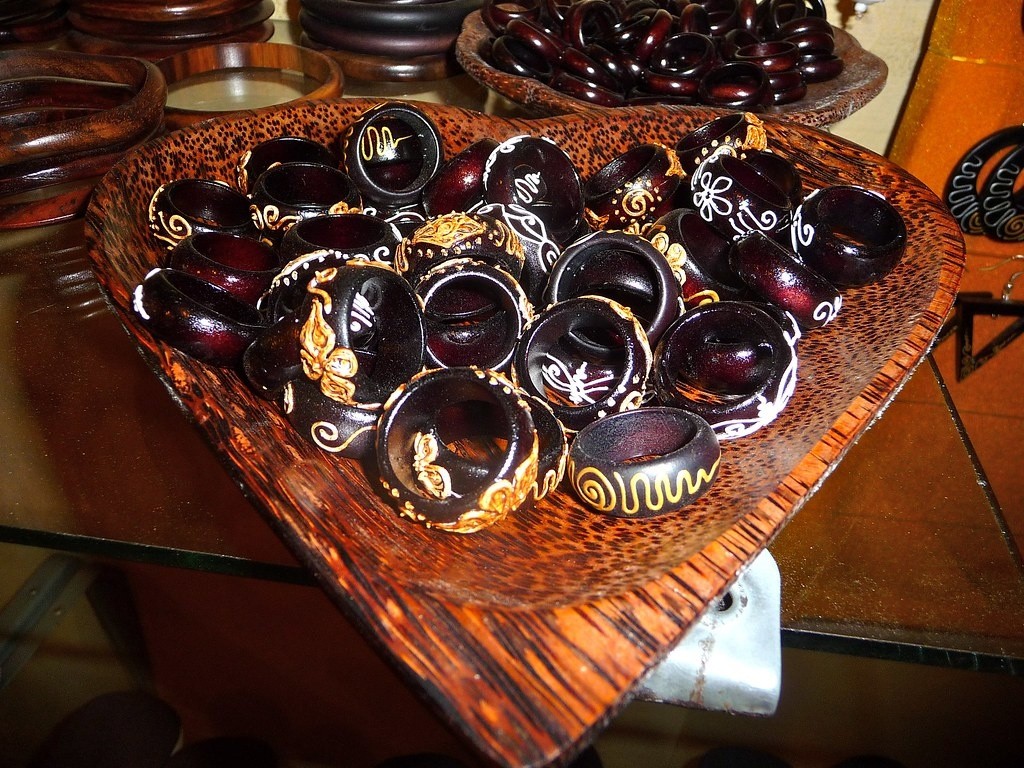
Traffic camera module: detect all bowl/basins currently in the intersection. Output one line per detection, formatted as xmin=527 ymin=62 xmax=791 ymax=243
xmin=287 ymin=1 xmax=489 ymax=85
xmin=67 ymin=0 xmax=274 ymax=58
xmin=156 ymin=45 xmax=344 ymax=130
xmin=0 ymin=47 xmax=167 ymax=226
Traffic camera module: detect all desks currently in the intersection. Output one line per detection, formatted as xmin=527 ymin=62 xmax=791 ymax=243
xmin=0 ymin=0 xmax=1024 ymax=676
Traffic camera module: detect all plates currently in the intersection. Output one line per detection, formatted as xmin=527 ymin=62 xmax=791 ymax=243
xmin=457 ymin=2 xmax=889 ymax=132
xmin=81 ymin=97 xmax=965 ymax=768
